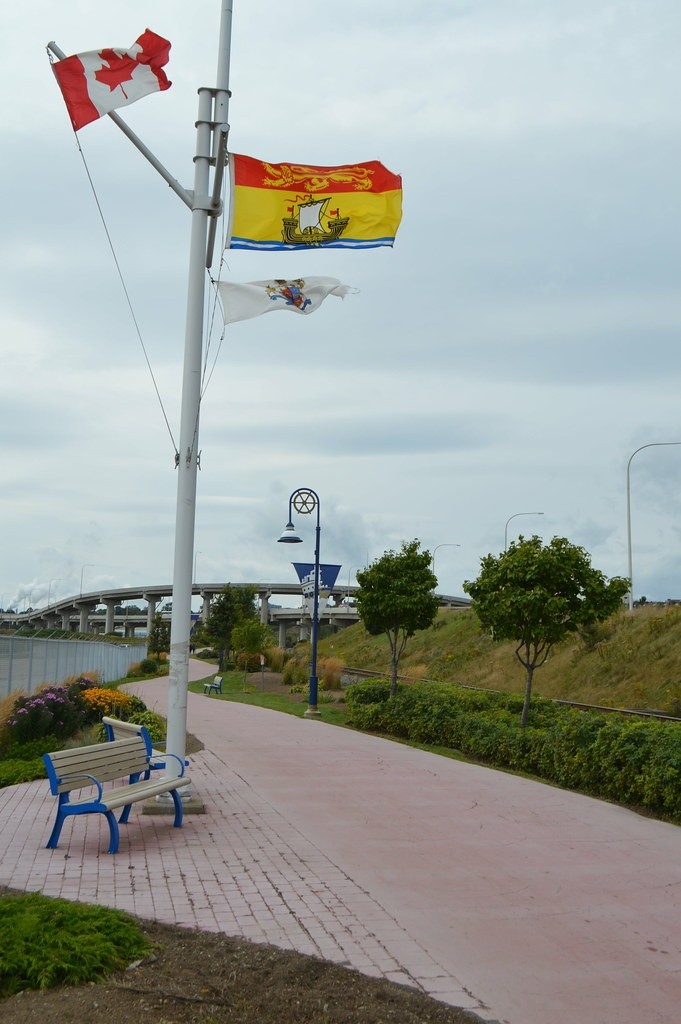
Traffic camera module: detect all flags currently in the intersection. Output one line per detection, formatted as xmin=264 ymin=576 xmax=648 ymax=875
xmin=226 ymin=152 xmax=402 ymax=251
xmin=215 ymin=276 xmax=349 ymax=325
xmin=51 ymin=28 xmax=171 ymax=132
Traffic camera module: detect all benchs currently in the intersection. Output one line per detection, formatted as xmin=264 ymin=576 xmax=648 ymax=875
xmin=203 ymin=675 xmax=223 ymax=695
xmin=43 ymin=737 xmax=192 ymax=853
xmin=102 ymin=716 xmax=190 ymax=781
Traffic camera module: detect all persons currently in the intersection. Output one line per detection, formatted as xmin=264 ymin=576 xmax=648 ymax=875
xmin=189 ymin=644 xmax=196 ymax=654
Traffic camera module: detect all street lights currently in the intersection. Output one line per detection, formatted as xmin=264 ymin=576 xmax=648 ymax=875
xmin=432 ymin=545 xmax=462 ymax=598
xmin=80 ymin=564 xmax=95 ymax=594
xmin=275 ymin=488 xmax=322 ymax=718
xmin=193 ymin=551 xmax=203 ymax=583
xmin=48 ymin=579 xmax=63 ymax=606
xmin=504 ymin=511 xmax=542 ymax=553
